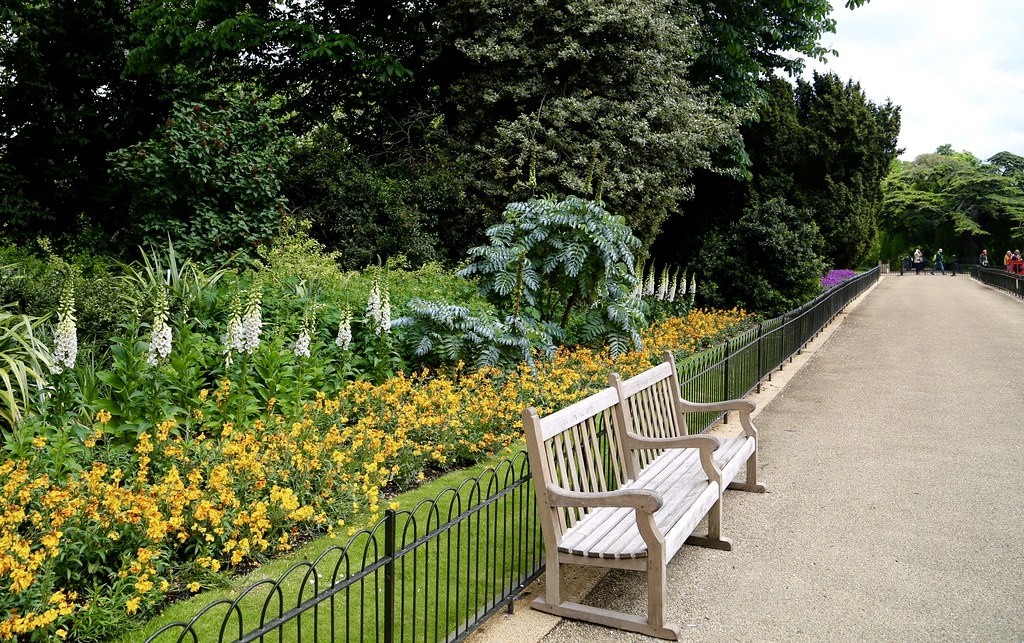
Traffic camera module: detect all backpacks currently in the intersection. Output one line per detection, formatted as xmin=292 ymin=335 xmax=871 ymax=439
xmin=933 ymin=254 xmax=937 ymax=262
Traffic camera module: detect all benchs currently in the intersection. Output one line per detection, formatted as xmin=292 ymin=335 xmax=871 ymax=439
xmin=521 ymin=349 xmax=766 ymax=641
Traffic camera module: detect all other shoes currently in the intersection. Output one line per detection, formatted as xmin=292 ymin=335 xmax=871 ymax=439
xmin=943 ymin=273 xmax=946 ymax=275
xmin=930 ymin=272 xmax=934 ymax=275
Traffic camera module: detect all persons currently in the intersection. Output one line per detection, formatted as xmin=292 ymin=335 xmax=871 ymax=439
xmin=1004 ymin=249 xmax=1024 ymax=274
xmin=914 ymin=248 xmax=923 ymax=274
xmin=980 ymin=249 xmax=990 ymax=268
xmin=931 ymin=249 xmax=947 ymax=275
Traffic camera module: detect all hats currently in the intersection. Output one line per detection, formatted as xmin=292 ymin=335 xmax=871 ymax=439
xmin=982 ymin=250 xmax=987 ymax=254
